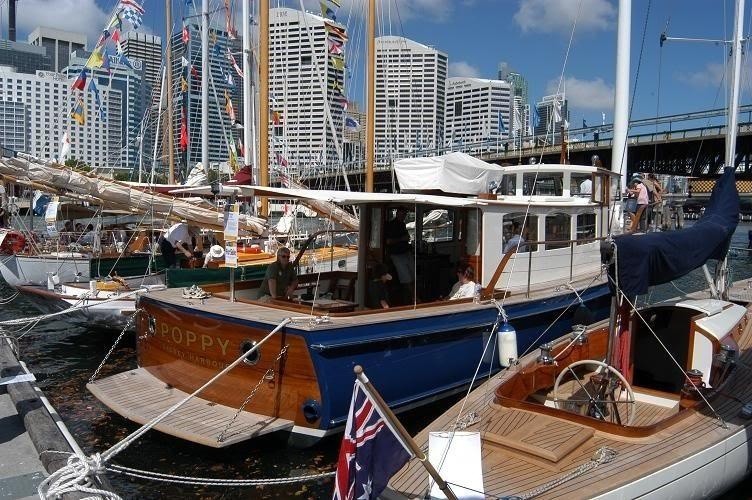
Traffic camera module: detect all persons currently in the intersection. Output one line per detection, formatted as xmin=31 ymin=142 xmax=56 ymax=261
xmin=648 ymin=174 xmax=663 ymax=205
xmin=503 ymin=224 xmax=529 ymax=252
xmin=382 ymin=205 xmax=421 ymax=307
xmin=629 ymin=177 xmax=648 ymax=233
xmin=75 ymin=222 xmax=85 ymax=242
xmin=442 ymin=263 xmax=479 ymax=301
xmin=83 ymin=223 xmax=97 ymax=243
xmin=256 ymin=247 xmax=298 ymax=299
xmin=160 ymin=219 xmax=199 ymax=271
xmin=60 ymin=221 xmax=76 ymax=243
xmin=203 ymin=244 xmax=227 ymax=269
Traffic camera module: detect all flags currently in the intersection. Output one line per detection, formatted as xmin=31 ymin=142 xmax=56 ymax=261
xmin=514 ymin=108 xmax=521 ymax=130
xmin=499 ymin=112 xmax=505 ymax=133
xmin=554 ymin=99 xmax=562 ymax=122
xmin=583 ymin=119 xmax=587 ymax=136
xmin=58 ymin=0 xmax=360 ymax=216
xmin=565 ymin=120 xmax=570 ymax=128
xmin=330 ymin=379 xmax=411 ymax=500
xmin=533 ymin=103 xmax=541 ymax=127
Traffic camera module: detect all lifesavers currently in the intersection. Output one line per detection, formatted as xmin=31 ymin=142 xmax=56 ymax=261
xmin=2 ymin=231 xmax=25 ymax=255
xmin=235 ymin=243 xmax=261 ymax=254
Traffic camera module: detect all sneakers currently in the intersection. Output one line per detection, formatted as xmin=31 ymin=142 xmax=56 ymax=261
xmin=182 ymin=284 xmax=212 ymax=299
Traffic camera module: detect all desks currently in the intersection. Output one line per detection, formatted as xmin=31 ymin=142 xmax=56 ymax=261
xmin=269 ymin=300 xmax=359 ymax=314
xmin=295 ymin=282 xmax=320 ymax=295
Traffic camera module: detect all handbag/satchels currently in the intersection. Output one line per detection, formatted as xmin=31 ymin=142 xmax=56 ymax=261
xmin=624 ymin=194 xmax=637 ymax=213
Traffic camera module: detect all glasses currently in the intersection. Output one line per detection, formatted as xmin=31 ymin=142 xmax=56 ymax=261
xmin=278 ymin=255 xmax=290 ymax=259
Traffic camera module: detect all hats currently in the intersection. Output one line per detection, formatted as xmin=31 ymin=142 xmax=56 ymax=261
xmin=209 ymin=244 xmax=225 ymax=258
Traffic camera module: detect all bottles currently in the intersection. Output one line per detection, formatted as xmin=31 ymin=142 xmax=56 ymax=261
xmin=473 ymin=281 xmax=482 ymax=304
